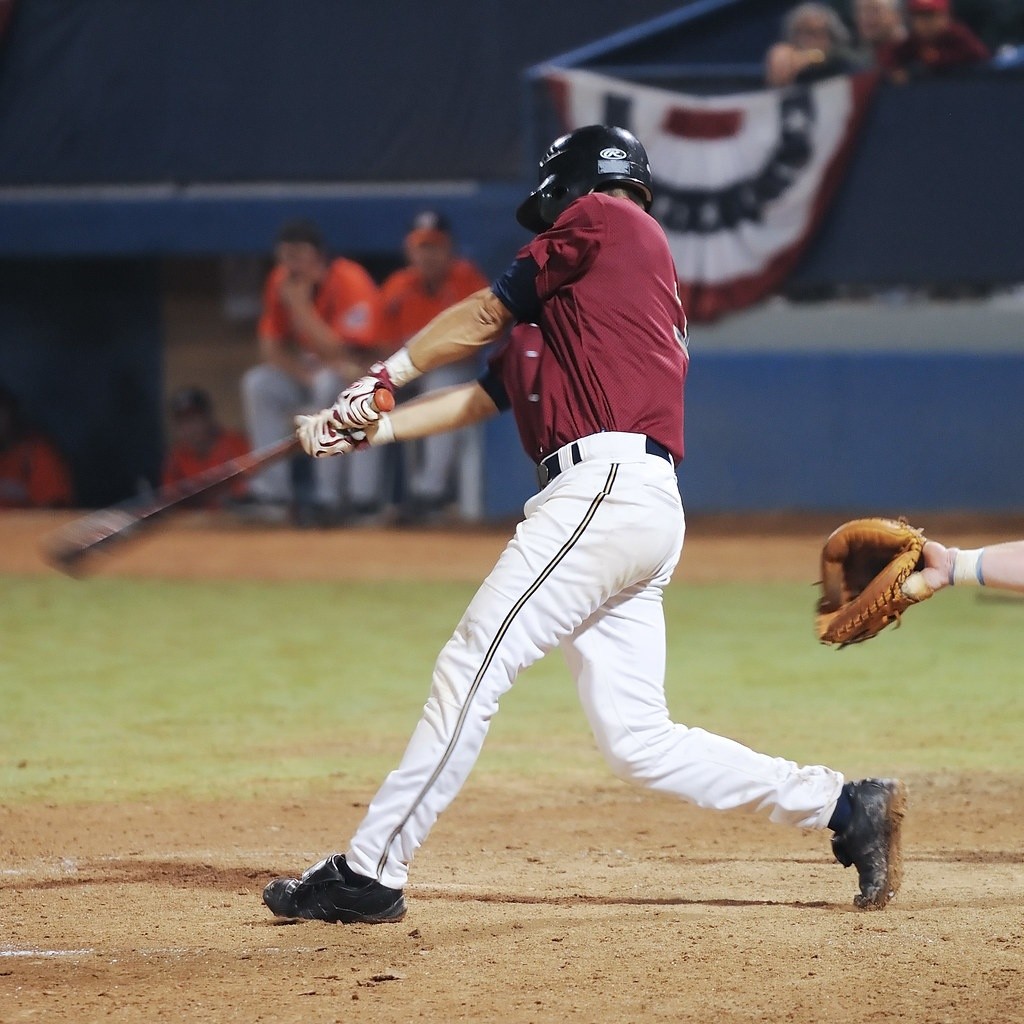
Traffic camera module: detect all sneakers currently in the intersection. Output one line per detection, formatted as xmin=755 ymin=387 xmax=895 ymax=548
xmin=263 ymin=855 xmax=408 ymax=925
xmin=830 ymin=778 xmax=907 ymax=912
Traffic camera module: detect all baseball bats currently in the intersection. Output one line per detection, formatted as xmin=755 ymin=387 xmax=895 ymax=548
xmin=35 ymin=388 xmax=395 ymax=580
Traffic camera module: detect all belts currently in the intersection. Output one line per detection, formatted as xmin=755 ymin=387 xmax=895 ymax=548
xmin=537 ymin=435 xmax=672 ymax=491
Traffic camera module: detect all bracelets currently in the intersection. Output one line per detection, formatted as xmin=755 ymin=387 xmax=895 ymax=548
xmin=951 ymin=548 xmax=987 ymax=589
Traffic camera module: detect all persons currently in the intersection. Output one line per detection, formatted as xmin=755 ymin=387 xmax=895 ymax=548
xmin=264 ymin=123 xmax=905 ymax=954
xmin=766 ymin=0 xmax=986 ymax=138
xmin=814 ymin=516 xmax=1022 ymax=648
xmin=226 ymin=211 xmax=500 ymax=530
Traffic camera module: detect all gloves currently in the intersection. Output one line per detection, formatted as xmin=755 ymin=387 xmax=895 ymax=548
xmin=329 ymin=359 xmax=403 ymax=431
xmin=297 ymin=401 xmax=395 ymax=458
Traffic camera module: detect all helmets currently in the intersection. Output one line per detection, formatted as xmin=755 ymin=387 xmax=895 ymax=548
xmin=516 ymin=124 xmax=654 ymax=234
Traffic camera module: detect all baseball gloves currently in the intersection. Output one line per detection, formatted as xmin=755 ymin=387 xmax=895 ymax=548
xmin=814 ymin=516 xmax=936 ymax=651
